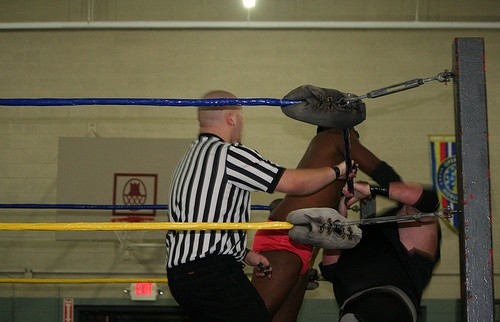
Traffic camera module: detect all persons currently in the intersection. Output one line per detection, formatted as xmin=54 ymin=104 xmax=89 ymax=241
xmin=165 ymin=91 xmax=358 ymax=322
xmin=317 ymin=183 xmax=442 ymax=322
xmin=250 ymin=126 xmax=420 ymax=322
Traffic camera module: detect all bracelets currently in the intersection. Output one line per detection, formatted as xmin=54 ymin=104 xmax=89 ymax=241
xmin=329 ymin=166 xmax=340 ymax=178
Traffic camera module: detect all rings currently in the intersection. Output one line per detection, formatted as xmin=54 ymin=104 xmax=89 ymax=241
xmin=260 ymin=267 xmax=267 ymax=272
xmin=257 ymin=262 xmax=263 ymax=268
xmin=264 ymin=272 xmax=271 ymax=276
xmin=265 ymin=263 xmax=272 ymax=270
xmin=269 ymin=271 xmax=273 ymax=275
